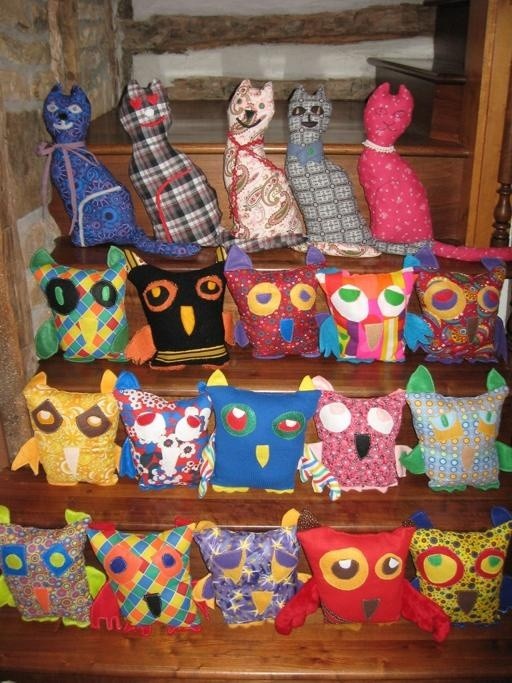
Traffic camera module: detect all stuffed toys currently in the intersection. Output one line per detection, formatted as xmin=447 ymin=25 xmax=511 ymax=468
xmin=313 ymin=252 xmax=435 ymax=364
xmin=223 ymin=243 xmax=329 ymax=360
xmin=191 ymin=507 xmax=312 ymax=629
xmin=357 ymin=81 xmax=511 ymax=262
xmin=197 ymin=369 xmax=342 ymax=502
xmin=410 ymin=506 xmax=512 ymax=628
xmin=275 ymin=510 xmax=451 ymax=643
xmin=36 ymin=82 xmax=202 ymax=257
xmin=122 ymin=243 xmax=227 ymax=371
xmin=284 ymin=83 xmax=436 ymax=256
xmin=117 ymin=77 xmax=309 ymax=253
xmin=11 ymin=369 xmax=122 ymax=486
xmin=113 ymin=369 xmax=212 ymax=490
xmin=414 ymin=243 xmax=507 ymax=365
xmin=298 ymin=375 xmax=413 ymax=493
xmin=399 ymin=363 xmax=512 ymax=493
xmin=29 ymin=245 xmax=131 ymax=362
xmin=223 ymin=77 xmax=382 ymax=258
xmin=88 ymin=516 xmax=210 ymax=636
xmin=0 ymin=504 xmax=107 ymax=629
